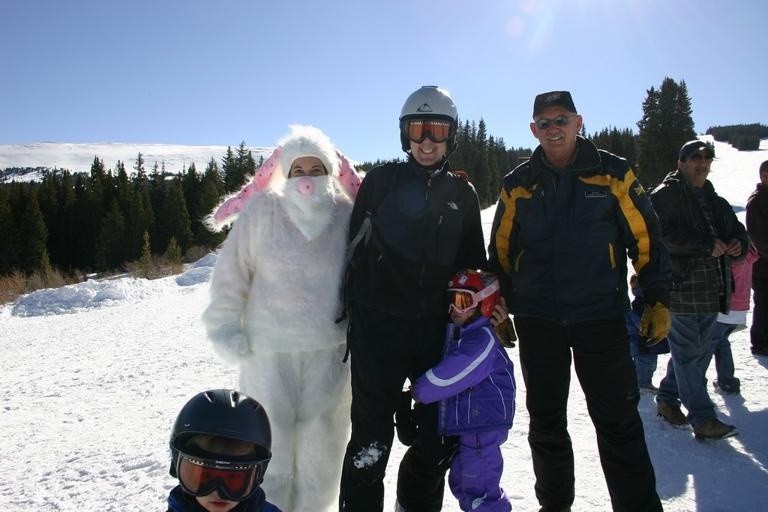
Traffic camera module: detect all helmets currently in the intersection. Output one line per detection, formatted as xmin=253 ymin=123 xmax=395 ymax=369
xmin=448 ymin=268 xmax=500 ymax=317
xmin=399 ymin=86 xmax=457 ymax=151
xmin=169 ymin=389 xmax=272 ymax=484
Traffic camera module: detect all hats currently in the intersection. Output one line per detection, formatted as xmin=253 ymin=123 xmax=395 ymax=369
xmin=533 ymin=91 xmax=577 ymax=117
xmin=679 ymin=140 xmax=712 ymax=161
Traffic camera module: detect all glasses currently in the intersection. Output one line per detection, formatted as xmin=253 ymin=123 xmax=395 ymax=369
xmin=536 ymin=116 xmax=567 ymax=129
xmin=446 ymin=288 xmax=478 ymax=314
xmin=403 ymin=119 xmax=454 ymax=143
xmin=176 ymin=451 xmax=264 ymax=502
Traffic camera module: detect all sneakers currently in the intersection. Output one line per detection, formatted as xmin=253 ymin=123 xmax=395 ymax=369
xmin=658 ymin=404 xmax=690 ymax=429
xmin=694 ymin=419 xmax=739 ymax=440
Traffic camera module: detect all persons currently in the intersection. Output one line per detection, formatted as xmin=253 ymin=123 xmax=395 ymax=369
xmin=199 ymin=124 xmax=370 ymax=512
xmin=621 ymin=273 xmax=666 ymax=390
xmin=338 ymin=82 xmax=508 ymax=512
xmin=712 ymin=226 xmax=760 ymax=395
xmin=163 ymin=389 xmax=283 ymax=512
xmin=407 ymin=264 xmax=516 ymax=511
xmin=745 ymin=160 xmax=768 ymax=359
xmin=489 ymin=91 xmax=672 ymax=512
xmin=652 ymin=139 xmax=749 ymax=441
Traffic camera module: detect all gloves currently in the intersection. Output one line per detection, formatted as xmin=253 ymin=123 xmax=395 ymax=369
xmin=495 ymin=317 xmax=517 ymax=348
xmin=639 ymin=302 xmax=671 ymax=347
